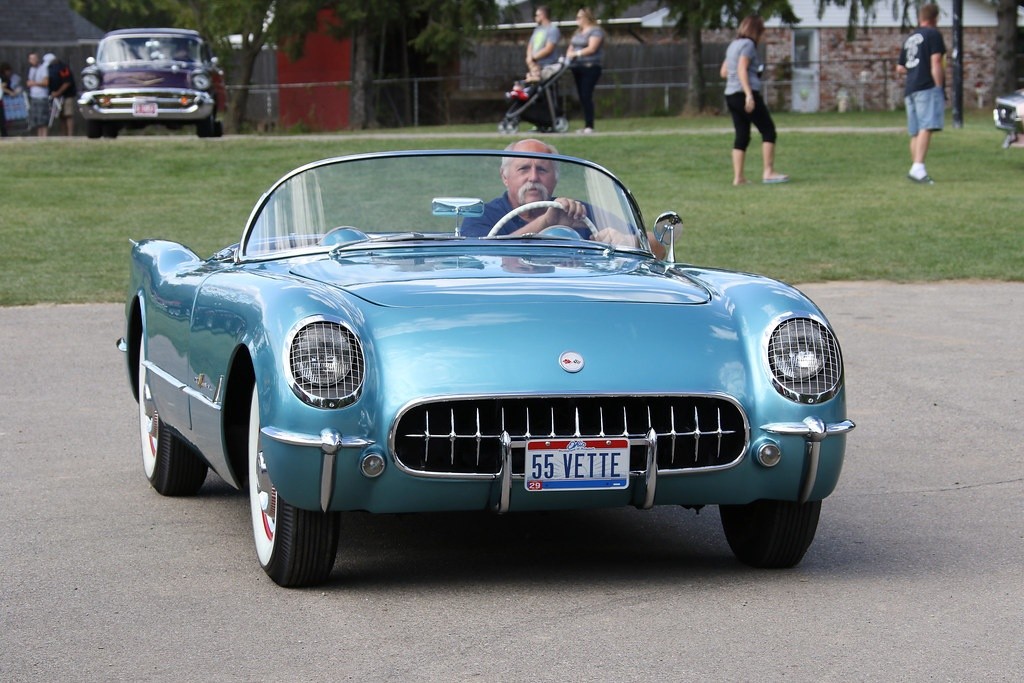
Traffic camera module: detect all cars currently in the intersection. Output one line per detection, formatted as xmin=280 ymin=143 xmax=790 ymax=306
xmin=76 ymin=28 xmax=224 ymax=138
xmin=114 ymin=146 xmax=855 ymax=591
xmin=992 ymin=87 xmax=1023 ymax=148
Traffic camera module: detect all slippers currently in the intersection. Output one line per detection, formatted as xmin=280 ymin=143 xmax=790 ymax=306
xmin=907 ymin=174 xmax=936 ymax=185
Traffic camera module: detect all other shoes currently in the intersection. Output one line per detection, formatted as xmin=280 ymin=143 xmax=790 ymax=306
xmin=576 ymin=127 xmax=593 ymax=135
xmin=733 ymin=177 xmax=753 ymax=186
xmin=761 ymin=173 xmax=790 ymax=184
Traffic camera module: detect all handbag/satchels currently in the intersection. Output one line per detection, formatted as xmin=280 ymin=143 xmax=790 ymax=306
xmin=2 ymin=91 xmax=29 ymax=121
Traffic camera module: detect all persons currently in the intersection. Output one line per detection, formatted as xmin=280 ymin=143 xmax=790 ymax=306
xmin=174 ymin=42 xmax=196 ymax=62
xmin=720 ymin=13 xmax=789 ymax=186
xmin=566 ymin=6 xmax=606 ymax=134
xmin=461 ymin=139 xmax=666 ymax=260
xmin=896 ymin=4 xmax=948 ymax=184
xmin=43 ymin=53 xmax=76 ymax=136
xmin=0 ymin=65 xmax=24 ymax=137
xmin=525 ymin=5 xmax=561 ymax=132
xmin=26 ymin=53 xmax=49 ymax=136
xmin=506 ymin=64 xmax=542 ymax=101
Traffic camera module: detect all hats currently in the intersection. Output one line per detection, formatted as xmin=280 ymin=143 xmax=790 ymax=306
xmin=40 ymin=53 xmax=56 ymax=68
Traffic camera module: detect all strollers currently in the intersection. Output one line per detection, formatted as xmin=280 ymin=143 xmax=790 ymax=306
xmin=497 ymin=60 xmax=569 ymax=134
